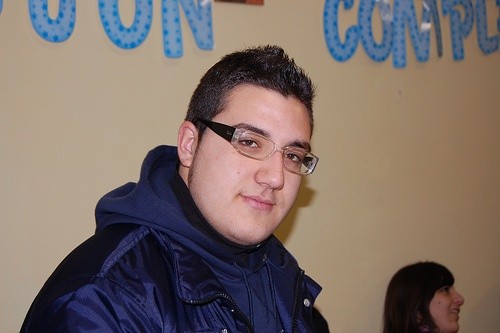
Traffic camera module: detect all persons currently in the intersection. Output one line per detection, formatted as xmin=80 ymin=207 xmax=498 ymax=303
xmin=382 ymin=261 xmax=464 ymax=333
xmin=19 ymin=45 xmax=329 ymax=333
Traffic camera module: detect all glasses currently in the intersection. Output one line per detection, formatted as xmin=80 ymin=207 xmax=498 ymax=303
xmin=202 ymin=120 xmax=319 ymax=176
